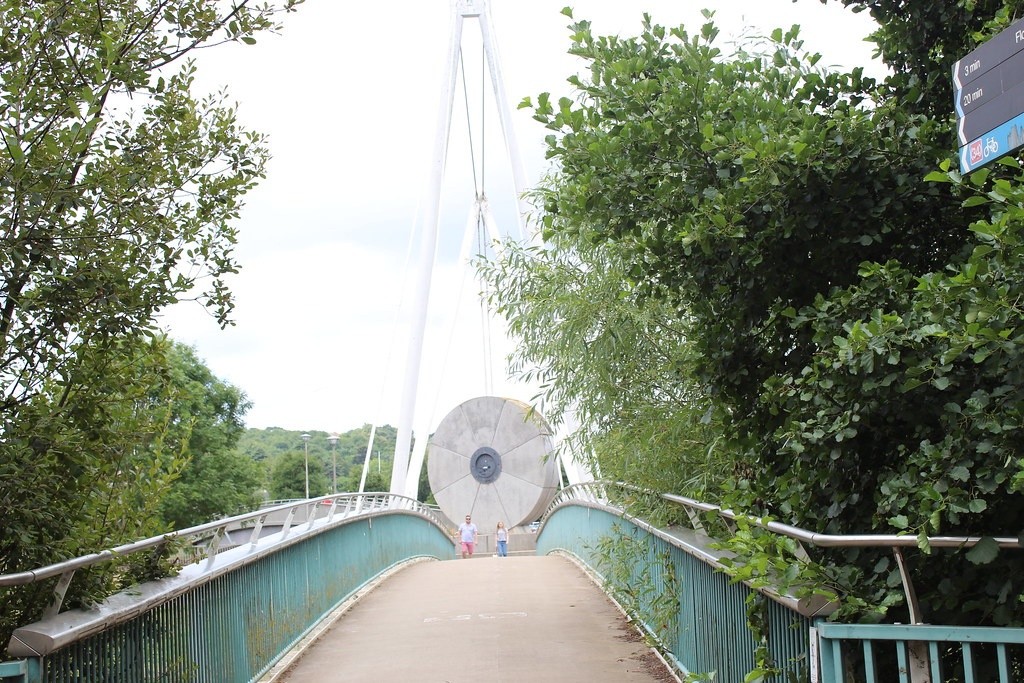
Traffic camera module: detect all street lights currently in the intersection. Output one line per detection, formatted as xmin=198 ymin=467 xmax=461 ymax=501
xmin=300 ymin=433 xmax=312 ymax=499
xmin=326 ymin=435 xmax=341 ymax=494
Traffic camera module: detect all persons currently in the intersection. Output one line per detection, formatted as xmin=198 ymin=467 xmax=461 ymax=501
xmin=458 ymin=515 xmax=478 ymax=559
xmin=495 ymin=522 xmax=509 ymax=558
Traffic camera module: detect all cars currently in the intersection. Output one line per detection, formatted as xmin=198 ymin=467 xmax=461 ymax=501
xmin=527 ymin=521 xmax=539 ymax=533
